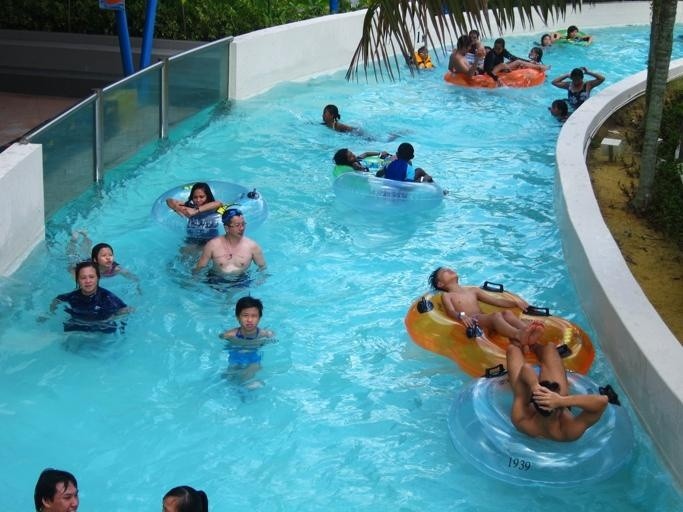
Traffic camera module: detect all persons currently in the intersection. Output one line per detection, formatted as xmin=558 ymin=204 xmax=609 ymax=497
xmin=429 ymin=264 xmax=545 ymax=350
xmin=404 ymin=23 xmax=606 ymax=123
xmin=374 ymin=143 xmax=450 ymax=204
xmin=60 ymin=226 xmax=141 ymax=290
xmin=332 ymin=148 xmax=392 ymax=173
xmin=165 ymin=182 xmax=224 ymax=255
xmin=288 ymin=102 xmax=416 ymax=147
xmin=34 ymin=466 xmax=80 ymax=512
xmin=35 ymin=256 xmax=137 ymax=337
xmin=161 ymin=484 xmax=209 ymax=512
xmin=190 ymin=208 xmax=269 ymax=289
xmin=505 ymin=338 xmax=610 ymax=444
xmin=211 ymin=294 xmax=276 ymax=380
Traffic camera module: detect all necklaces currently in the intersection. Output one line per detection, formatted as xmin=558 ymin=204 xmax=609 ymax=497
xmin=227 ymin=252 xmax=233 ymax=261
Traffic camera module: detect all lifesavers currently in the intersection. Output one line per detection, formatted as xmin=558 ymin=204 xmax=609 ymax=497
xmin=552 ymin=29 xmax=590 ymax=47
xmin=404 ymin=281 xmax=595 ymax=377
xmin=333 ymin=172 xmax=444 ymax=210
xmin=445 ymin=362 xmax=634 ymax=487
xmin=445 ymin=68 xmax=545 ymax=87
xmin=152 ymin=180 xmax=267 ymax=238
xmin=333 ymin=154 xmax=413 ymax=178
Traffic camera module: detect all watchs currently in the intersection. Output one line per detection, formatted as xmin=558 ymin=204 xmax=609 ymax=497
xmin=457 ymin=310 xmax=467 ymax=321
